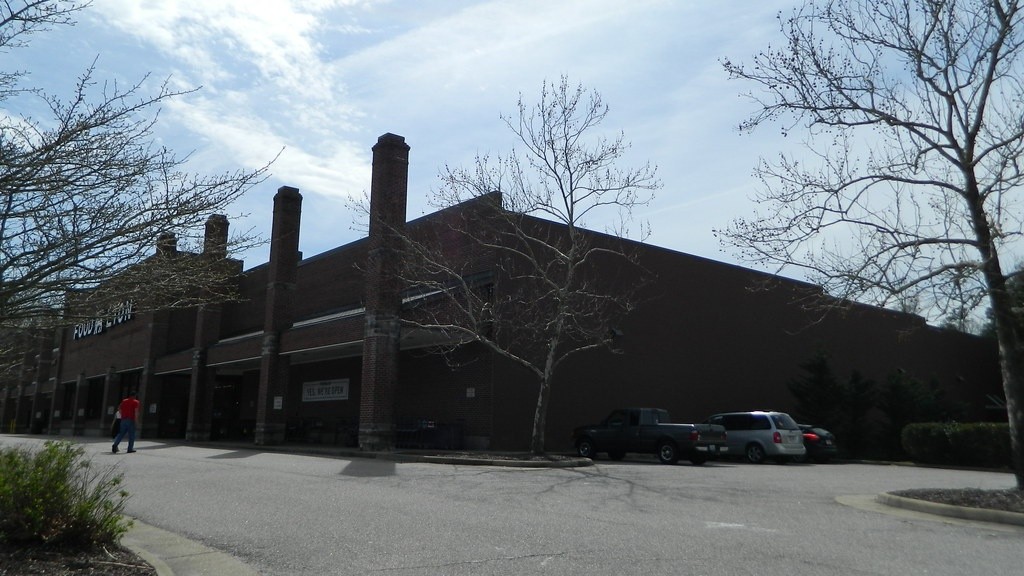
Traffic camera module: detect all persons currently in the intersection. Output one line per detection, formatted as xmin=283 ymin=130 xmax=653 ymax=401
xmin=111 ymin=404 xmax=123 ymax=439
xmin=112 ymin=390 xmax=141 ymax=454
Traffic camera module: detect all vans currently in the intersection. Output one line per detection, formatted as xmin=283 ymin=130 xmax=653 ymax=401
xmin=698 ymin=411 xmax=808 ymax=462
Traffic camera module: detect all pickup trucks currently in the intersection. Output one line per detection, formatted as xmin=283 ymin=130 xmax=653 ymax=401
xmin=571 ymin=407 xmax=730 ymax=467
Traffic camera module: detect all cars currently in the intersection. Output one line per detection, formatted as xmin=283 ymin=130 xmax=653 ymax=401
xmin=792 ymin=423 xmax=839 ymax=463
